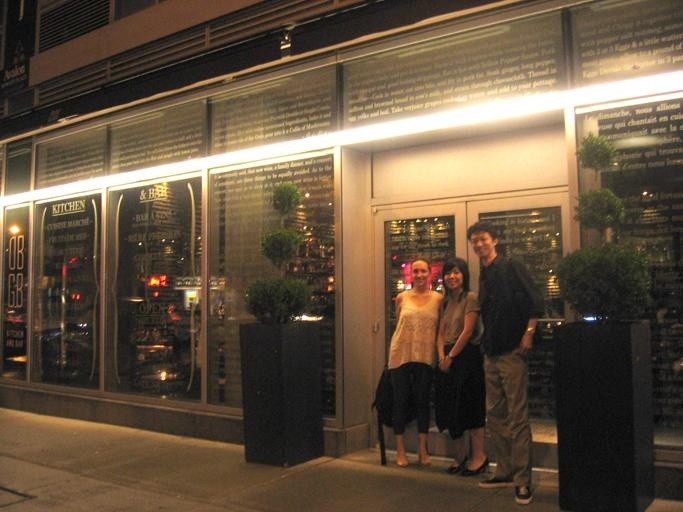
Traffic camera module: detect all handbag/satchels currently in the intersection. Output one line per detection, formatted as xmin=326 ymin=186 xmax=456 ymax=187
xmin=514 ymin=320 xmax=543 ymax=348
xmin=435 ymin=362 xmax=463 ymax=439
xmin=375 ymin=364 xmax=417 ymax=428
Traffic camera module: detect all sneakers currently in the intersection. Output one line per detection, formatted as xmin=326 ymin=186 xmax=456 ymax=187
xmin=515 ymin=483 xmax=532 ymax=505
xmin=479 ymin=475 xmax=514 ymax=488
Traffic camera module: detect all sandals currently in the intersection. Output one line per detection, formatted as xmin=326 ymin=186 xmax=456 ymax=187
xmin=396 ymin=451 xmax=408 ymax=468
xmin=419 ymin=451 xmax=431 ymax=467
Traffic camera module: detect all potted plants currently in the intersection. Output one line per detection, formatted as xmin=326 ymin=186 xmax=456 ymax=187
xmin=237 ymin=183 xmax=326 ymax=468
xmin=551 ymin=132 xmax=656 ymax=512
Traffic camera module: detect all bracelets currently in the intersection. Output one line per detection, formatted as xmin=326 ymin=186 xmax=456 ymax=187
xmin=526 ymin=328 xmax=536 ymax=335
xmin=446 ymin=353 xmax=455 ymax=361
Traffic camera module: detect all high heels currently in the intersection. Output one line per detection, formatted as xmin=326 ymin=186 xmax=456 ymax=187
xmin=461 ymin=456 xmax=489 ymax=476
xmin=447 ymin=454 xmax=468 ymax=474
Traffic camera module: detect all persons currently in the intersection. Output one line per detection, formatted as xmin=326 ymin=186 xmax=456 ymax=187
xmin=434 ymin=257 xmax=491 ymax=477
xmin=466 ymin=221 xmax=543 ymax=506
xmin=387 ymin=256 xmax=445 ymax=466
